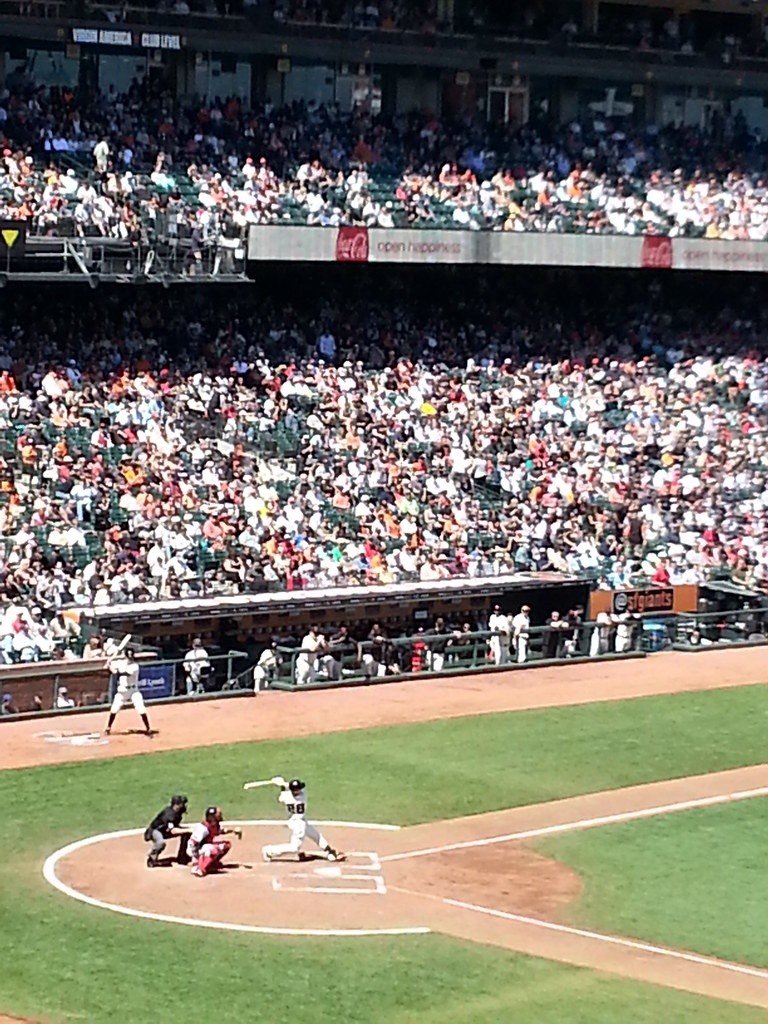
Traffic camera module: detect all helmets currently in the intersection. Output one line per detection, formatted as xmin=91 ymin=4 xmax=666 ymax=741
xmin=172 ymin=795 xmax=188 ymax=804
xmin=125 ymin=648 xmax=134 ymax=659
xmin=289 ymin=780 xmax=306 ymax=791
xmin=206 ymin=807 xmax=217 ymax=817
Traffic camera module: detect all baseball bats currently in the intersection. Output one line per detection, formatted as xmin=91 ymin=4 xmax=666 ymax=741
xmin=101 ymin=633 xmax=131 ymax=670
xmin=243 ymin=780 xmax=273 ymax=789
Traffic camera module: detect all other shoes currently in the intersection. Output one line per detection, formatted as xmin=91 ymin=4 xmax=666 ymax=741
xmin=262 ymin=846 xmax=271 ymax=862
xmin=328 ymin=851 xmax=347 ymax=862
xmin=147 ymin=857 xmax=154 ymax=867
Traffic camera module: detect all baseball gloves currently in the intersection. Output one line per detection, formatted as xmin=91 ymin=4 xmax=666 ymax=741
xmin=233 ymin=828 xmax=243 ymax=840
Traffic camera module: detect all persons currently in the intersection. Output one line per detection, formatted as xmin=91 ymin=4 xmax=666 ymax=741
xmin=0 ymin=0 xmax=768 ymax=735
xmin=144 ymin=796 xmax=192 ymax=866
xmin=261 ymin=775 xmax=347 ymax=862
xmin=186 ymin=806 xmax=242 ymax=877
